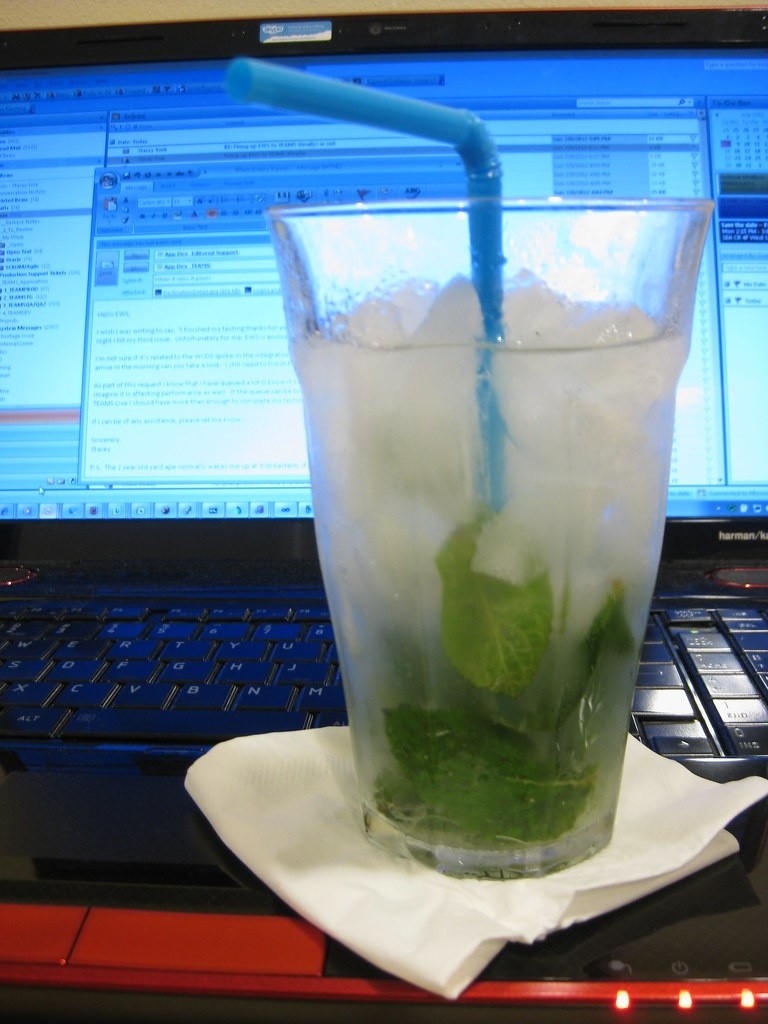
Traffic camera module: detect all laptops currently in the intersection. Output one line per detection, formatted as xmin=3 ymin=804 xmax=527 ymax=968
xmin=0 ymin=9 xmax=768 ymax=1024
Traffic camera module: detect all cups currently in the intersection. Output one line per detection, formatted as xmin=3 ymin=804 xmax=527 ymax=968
xmin=262 ymin=197 xmax=719 ymax=884
